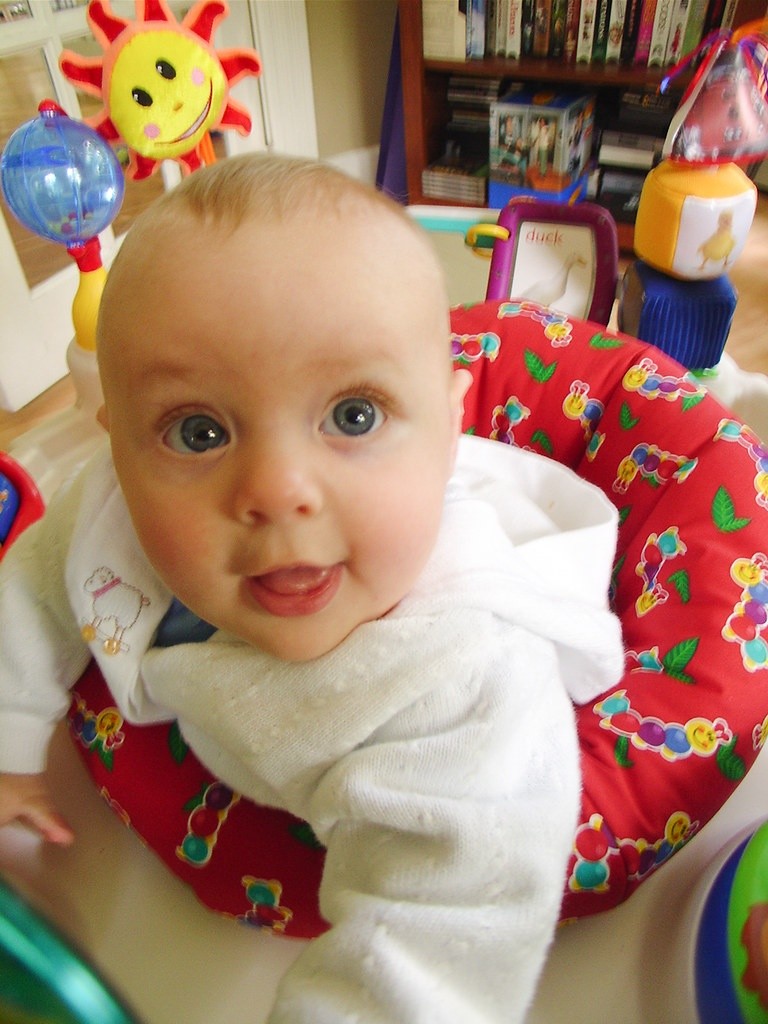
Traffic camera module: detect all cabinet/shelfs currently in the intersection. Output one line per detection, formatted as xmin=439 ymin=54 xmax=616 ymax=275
xmin=397 ymin=1 xmax=768 ymax=251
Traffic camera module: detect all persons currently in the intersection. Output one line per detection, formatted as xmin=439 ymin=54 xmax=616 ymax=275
xmin=0 ymin=151 xmax=626 ymax=1024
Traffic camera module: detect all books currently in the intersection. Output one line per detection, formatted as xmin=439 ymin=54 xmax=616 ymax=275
xmin=422 ymin=1 xmax=735 ymax=215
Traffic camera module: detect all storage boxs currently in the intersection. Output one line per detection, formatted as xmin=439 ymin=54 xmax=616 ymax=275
xmin=484 ymin=83 xmax=685 ymax=226
